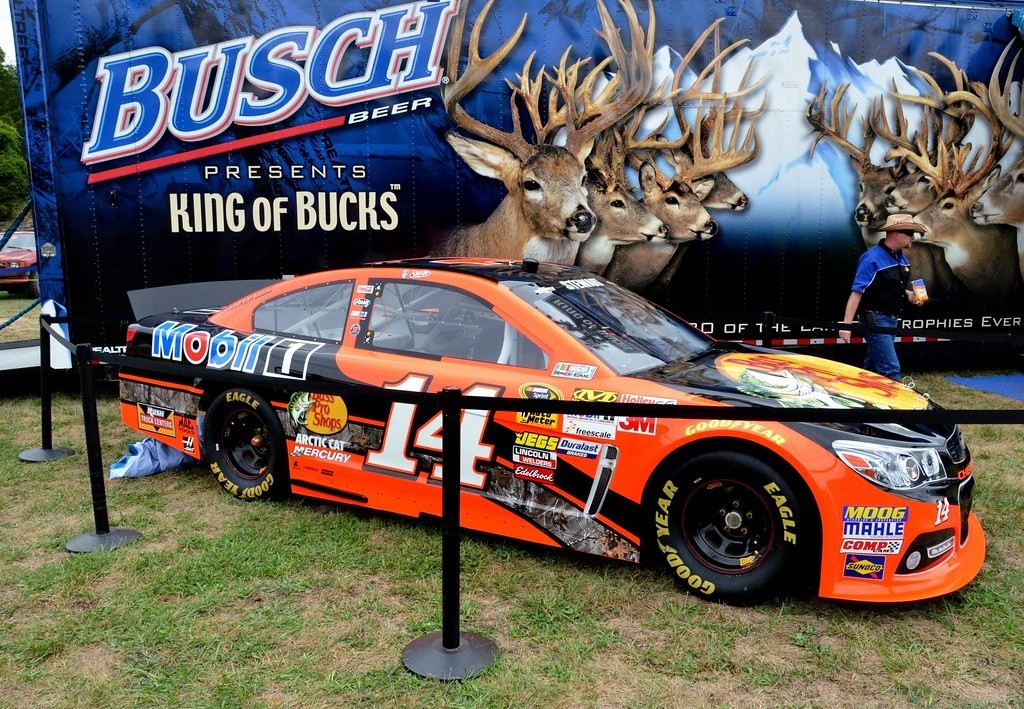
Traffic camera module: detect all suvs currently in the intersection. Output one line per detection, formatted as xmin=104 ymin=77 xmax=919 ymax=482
xmin=0 ymin=231 xmax=41 ymax=296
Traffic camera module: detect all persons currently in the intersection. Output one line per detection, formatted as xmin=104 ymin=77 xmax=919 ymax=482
xmin=838 ymin=213 xmax=929 ymax=380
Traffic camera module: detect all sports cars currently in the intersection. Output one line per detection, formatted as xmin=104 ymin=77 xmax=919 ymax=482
xmin=115 ymin=253 xmax=986 ymax=604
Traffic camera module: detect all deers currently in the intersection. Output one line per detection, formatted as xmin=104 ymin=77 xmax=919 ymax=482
xmin=427 ymin=0 xmax=772 ymax=288
xmin=804 ymin=35 xmax=1024 ymax=299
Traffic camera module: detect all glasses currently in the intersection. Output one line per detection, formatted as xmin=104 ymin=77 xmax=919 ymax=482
xmin=894 ymin=228 xmax=914 ymax=237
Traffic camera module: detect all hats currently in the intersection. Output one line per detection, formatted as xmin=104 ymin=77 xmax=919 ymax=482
xmin=879 ymin=213 xmax=927 ymax=234
